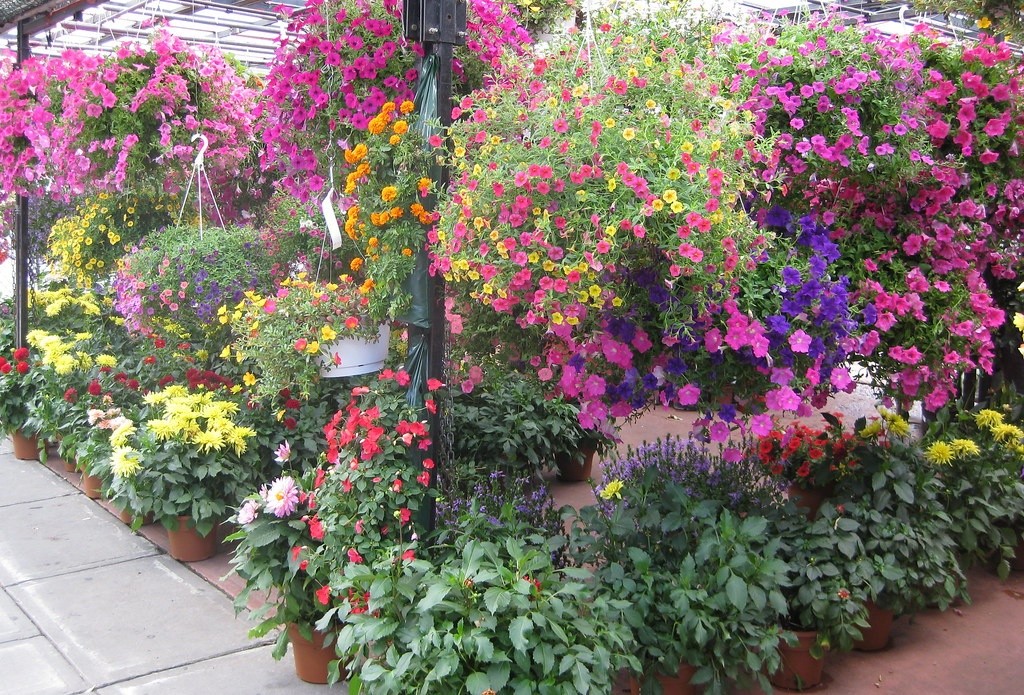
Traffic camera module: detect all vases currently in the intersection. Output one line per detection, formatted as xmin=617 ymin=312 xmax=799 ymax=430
xmin=788 ymin=486 xmax=820 ymax=519
xmin=287 ymin=621 xmax=350 ymax=684
xmin=851 ymin=598 xmax=892 ymax=651
xmin=555 ymin=441 xmax=594 ymax=481
xmin=119 ymin=507 xmax=154 ymax=524
xmin=80 ymin=463 xmax=102 ymax=498
xmin=630 ymin=662 xmax=704 ymax=695
xmin=14 ymin=430 xmax=47 ymax=460
xmin=167 ymin=516 xmax=217 ymax=562
xmin=765 ymin=631 xmax=823 ymax=689
xmin=1000 ymin=518 xmax=1024 ymax=570
xmin=63 ymin=455 xmax=76 ymax=471
xmin=312 ymin=320 xmax=390 ymax=378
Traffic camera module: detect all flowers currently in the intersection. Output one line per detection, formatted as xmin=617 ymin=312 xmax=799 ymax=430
xmin=0 ymin=0 xmax=1024 ymax=695
